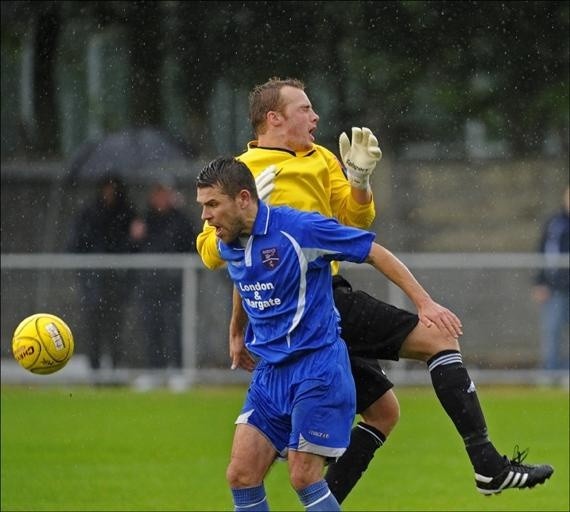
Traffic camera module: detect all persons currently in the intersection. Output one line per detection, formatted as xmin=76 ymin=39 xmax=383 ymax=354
xmin=131 ymin=171 xmax=185 ymax=373
xmin=192 ymin=78 xmax=554 ymax=512
xmin=535 ymin=178 xmax=569 ymax=380
xmin=71 ymin=173 xmax=136 ymax=377
xmin=195 ymin=154 xmax=462 ymax=512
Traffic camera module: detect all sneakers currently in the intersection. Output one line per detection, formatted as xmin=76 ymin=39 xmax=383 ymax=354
xmin=474 ymin=457 xmax=554 ymax=497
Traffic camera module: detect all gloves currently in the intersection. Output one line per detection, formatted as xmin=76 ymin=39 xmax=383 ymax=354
xmin=256 ymin=167 xmax=275 ymax=200
xmin=338 ymin=126 xmax=382 ymax=190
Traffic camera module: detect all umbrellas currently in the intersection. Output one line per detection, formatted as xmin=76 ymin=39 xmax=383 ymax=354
xmin=79 ymin=121 xmax=191 ymax=191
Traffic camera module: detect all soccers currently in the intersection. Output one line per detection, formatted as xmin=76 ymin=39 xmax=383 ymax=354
xmin=11 ymin=314 xmax=74 ymax=374
